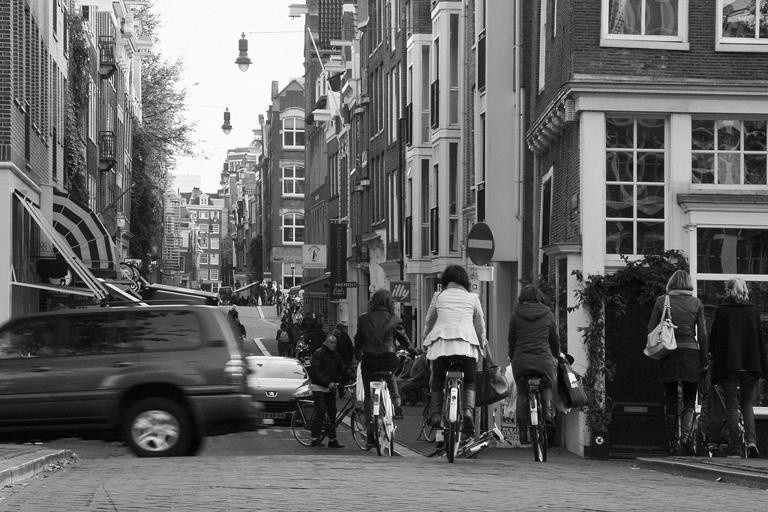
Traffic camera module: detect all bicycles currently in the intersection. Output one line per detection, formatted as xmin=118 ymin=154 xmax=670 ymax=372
xmin=287 ymin=338 xmax=576 ymax=464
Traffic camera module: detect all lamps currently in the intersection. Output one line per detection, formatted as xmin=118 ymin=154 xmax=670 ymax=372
xmin=221 ymin=106 xmax=233 ymax=135
xmin=233 ymin=32 xmax=253 ymax=72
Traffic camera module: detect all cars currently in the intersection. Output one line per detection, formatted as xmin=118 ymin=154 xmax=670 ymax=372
xmin=243 ymin=354 xmax=321 ymax=429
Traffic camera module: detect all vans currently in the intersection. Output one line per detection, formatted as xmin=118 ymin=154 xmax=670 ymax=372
xmin=0 ymin=302 xmax=268 ymax=458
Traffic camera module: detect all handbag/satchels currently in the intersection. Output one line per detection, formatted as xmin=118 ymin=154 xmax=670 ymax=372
xmin=475 ymin=365 xmax=510 ymax=406
xmin=558 ymin=362 xmax=584 ymax=407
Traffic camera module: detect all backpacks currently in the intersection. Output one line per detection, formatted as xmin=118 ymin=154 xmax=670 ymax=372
xmin=279 ymin=329 xmax=290 ymax=343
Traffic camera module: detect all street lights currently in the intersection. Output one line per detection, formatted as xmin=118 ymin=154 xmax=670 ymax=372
xmin=287 ymin=258 xmax=296 ymax=289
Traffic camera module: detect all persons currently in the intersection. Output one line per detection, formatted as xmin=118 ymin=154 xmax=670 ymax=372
xmin=509 ymin=285 xmax=564 ymax=441
xmin=648 ymin=270 xmax=708 ymax=454
xmin=218 ymin=276 xmax=352 ymax=398
xmin=352 ymin=289 xmax=420 ymax=446
xmin=420 ymin=265 xmax=487 ymax=430
xmin=708 ymin=277 xmax=768 ymax=458
xmin=311 ymin=335 xmax=347 ymax=448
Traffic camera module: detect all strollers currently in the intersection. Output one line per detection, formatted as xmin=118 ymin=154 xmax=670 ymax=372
xmin=698 ymin=355 xmax=749 ymax=458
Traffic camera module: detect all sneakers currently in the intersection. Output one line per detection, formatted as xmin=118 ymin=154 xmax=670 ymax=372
xmin=328 ymin=438 xmax=344 ymax=448
xmin=311 ymin=439 xmax=324 ymax=447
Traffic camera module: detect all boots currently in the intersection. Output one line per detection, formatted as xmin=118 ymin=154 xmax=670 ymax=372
xmin=430 ymin=391 xmax=444 ymax=426
xmin=542 ymin=398 xmax=555 ymax=430
xmin=366 ymin=420 xmax=374 ymax=443
xmin=518 ymin=423 xmax=528 ymax=443
xmin=393 ymin=397 xmax=402 ymax=415
xmin=463 ymin=389 xmax=476 ymax=434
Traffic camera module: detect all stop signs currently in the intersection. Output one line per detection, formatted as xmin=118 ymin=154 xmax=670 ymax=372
xmin=465 ymin=221 xmax=496 ymax=268
